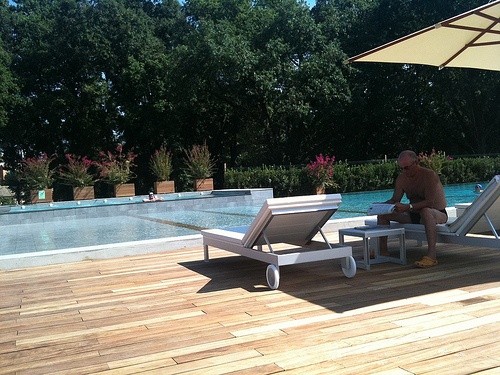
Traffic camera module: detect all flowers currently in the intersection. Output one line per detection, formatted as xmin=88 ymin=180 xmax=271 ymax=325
xmin=147 ymin=144 xmax=175 ymax=181
xmin=58 ymin=152 xmax=97 ymax=186
xmin=182 ymin=142 xmax=218 ymax=178
xmin=304 ymin=154 xmax=339 ymax=188
xmin=17 ymin=152 xmax=59 ymax=189
xmin=96 ymin=145 xmax=137 ymax=184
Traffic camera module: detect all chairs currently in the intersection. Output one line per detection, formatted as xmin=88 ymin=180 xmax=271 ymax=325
xmin=201 ymin=193 xmax=357 ymax=290
xmin=364 ymin=174 xmax=500 ymax=248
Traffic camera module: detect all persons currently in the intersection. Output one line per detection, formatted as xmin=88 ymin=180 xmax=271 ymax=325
xmin=363 ymin=150 xmax=448 ymax=267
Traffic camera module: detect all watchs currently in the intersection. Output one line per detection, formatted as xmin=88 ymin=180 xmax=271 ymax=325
xmin=409 ymin=203 xmax=413 ymax=213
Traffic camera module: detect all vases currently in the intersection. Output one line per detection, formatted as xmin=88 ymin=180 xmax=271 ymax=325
xmin=312 ymin=186 xmax=325 ymax=195
xmin=27 ymin=188 xmax=53 ymax=203
xmin=102 ymin=183 xmax=135 ymax=197
xmin=151 ymin=180 xmax=174 ymax=195
xmin=193 ymin=178 xmax=213 ymax=190
xmin=64 ymin=186 xmax=94 ymax=199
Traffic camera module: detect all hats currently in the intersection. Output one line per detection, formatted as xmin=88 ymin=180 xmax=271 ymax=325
xmin=476 ymin=184 xmax=483 ymax=189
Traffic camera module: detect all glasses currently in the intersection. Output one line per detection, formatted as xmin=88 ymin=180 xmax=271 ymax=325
xmin=399 ymin=161 xmax=416 ymax=173
xmin=475 ymin=187 xmax=480 ymax=188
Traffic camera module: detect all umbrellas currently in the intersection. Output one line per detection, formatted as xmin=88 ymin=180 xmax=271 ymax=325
xmin=344 ymin=0 xmax=500 ymax=72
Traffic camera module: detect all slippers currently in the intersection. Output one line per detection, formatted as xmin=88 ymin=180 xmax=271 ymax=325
xmin=362 ymin=251 xmax=390 ymax=259
xmin=414 ymin=256 xmax=438 ymax=268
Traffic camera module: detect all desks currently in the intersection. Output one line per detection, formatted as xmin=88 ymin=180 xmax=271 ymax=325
xmin=338 ymin=226 xmax=407 ymax=270
